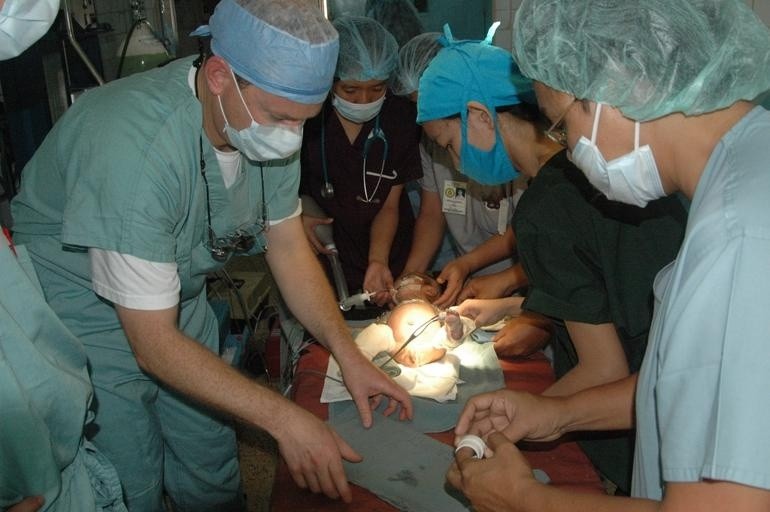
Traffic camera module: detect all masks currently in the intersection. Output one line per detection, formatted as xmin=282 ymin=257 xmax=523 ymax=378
xmin=459 ymin=142 xmax=520 ymax=187
xmin=334 ymin=96 xmax=385 ymax=124
xmin=572 ymin=101 xmax=667 ymax=209
xmin=0 ymin=0 xmax=60 ymax=60
xmin=217 ymin=71 xmax=305 ymax=163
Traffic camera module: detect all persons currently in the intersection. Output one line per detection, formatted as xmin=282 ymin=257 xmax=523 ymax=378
xmin=440 ymin=1 xmax=769 ymax=512
xmin=414 ymin=39 xmax=687 ymax=497
xmin=298 ymin=14 xmax=415 ymax=322
xmin=456 ymin=264 xmax=557 ymax=369
xmin=366 ymin=270 xmax=466 ymax=369
xmin=1 ymin=0 xmax=131 ymax=512
xmin=11 ymin=0 xmax=414 ymax=512
xmin=389 ymin=30 xmax=530 ymax=312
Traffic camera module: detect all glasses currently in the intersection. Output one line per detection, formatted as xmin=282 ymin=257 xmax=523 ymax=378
xmin=545 ymin=97 xmax=579 ymax=145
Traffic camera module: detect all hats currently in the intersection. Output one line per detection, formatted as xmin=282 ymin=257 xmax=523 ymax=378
xmin=206 ymin=1 xmax=337 ymax=104
xmin=415 ymin=43 xmax=531 ymax=124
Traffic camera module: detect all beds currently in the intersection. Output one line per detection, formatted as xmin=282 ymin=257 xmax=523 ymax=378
xmin=267 ymin=322 xmax=609 ymax=512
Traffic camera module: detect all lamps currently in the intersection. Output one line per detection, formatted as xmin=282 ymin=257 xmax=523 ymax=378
xmin=115 ymin=0 xmax=170 ymax=59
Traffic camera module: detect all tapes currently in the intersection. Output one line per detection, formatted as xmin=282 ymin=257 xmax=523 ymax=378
xmin=451 ymin=434 xmax=485 ymax=460
